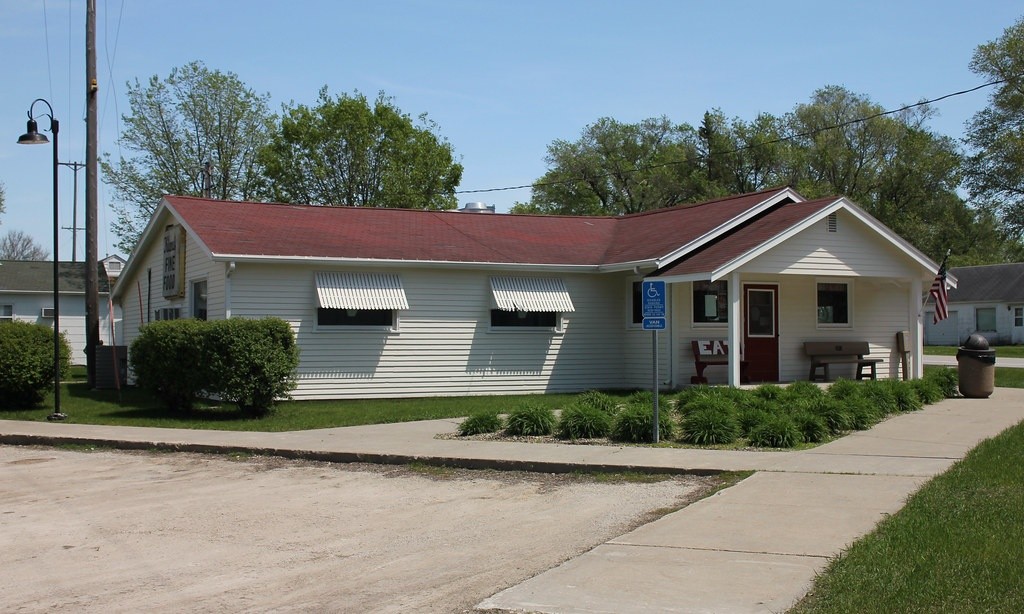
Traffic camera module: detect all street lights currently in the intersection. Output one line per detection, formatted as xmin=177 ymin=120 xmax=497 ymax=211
xmin=15 ymin=98 xmax=60 ymax=414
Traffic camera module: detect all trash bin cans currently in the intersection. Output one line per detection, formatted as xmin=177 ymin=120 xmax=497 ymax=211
xmin=95 ymin=346 xmax=127 ymax=390
xmin=957 ymin=334 xmax=996 ymax=398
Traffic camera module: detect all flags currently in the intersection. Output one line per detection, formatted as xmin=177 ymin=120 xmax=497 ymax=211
xmin=931 ymin=253 xmax=949 ymax=324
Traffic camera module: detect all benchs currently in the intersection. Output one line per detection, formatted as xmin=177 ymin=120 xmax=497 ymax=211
xmin=804 ymin=341 xmax=883 ymax=382
xmin=691 ymin=340 xmax=751 ymax=386
